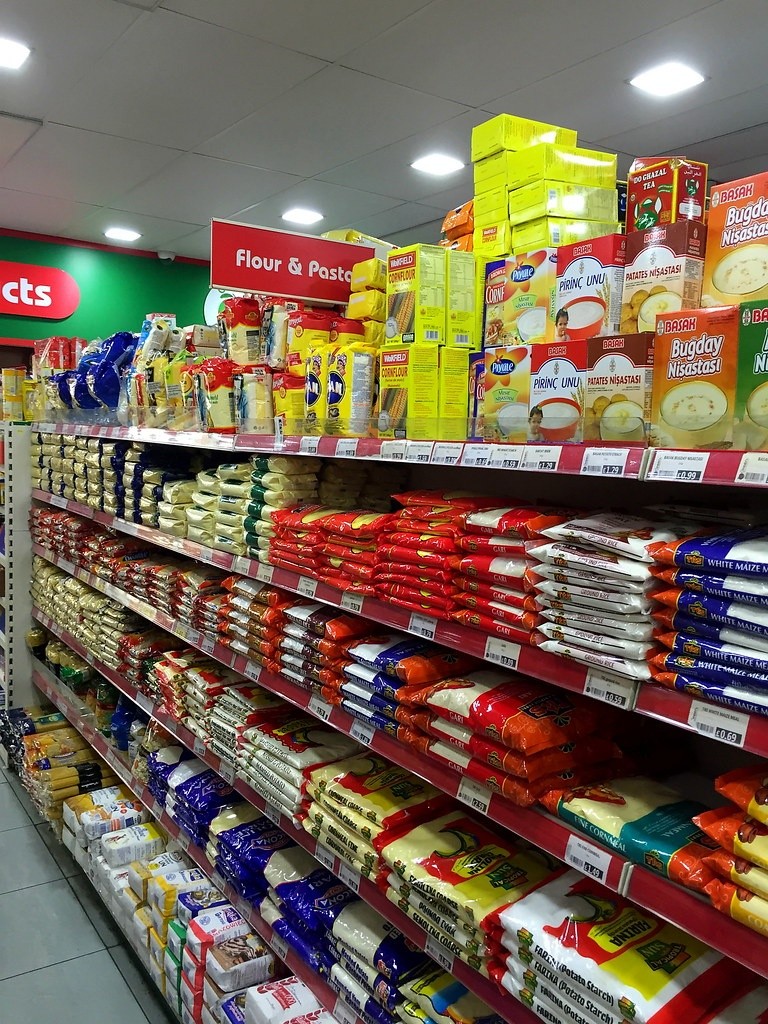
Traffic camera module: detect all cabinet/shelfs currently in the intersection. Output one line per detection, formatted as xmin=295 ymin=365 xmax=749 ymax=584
xmin=29 ymin=419 xmax=768 ymax=1024
xmin=0 ymin=421 xmax=49 ymax=769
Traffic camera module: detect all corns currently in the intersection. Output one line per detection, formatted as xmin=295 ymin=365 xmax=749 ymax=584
xmin=389 ymin=386 xmax=408 ymax=430
xmin=395 ymin=291 xmax=416 ymax=333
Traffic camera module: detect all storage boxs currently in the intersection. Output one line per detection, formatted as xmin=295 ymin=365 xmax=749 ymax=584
xmin=379 ymin=113 xmax=768 ymax=456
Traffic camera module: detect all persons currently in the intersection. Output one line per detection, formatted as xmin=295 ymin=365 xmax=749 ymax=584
xmin=529 ymin=405 xmax=547 ymax=440
xmin=194 ymin=375 xmax=207 ymax=426
xmin=313 ymin=355 xmax=346 ymax=376
xmin=218 ymin=319 xmax=228 ymax=360
xmin=554 ymin=309 xmax=570 ymax=341
xmin=259 ymin=305 xmax=276 ymax=355
xmin=234 ymin=374 xmax=248 ymax=424
